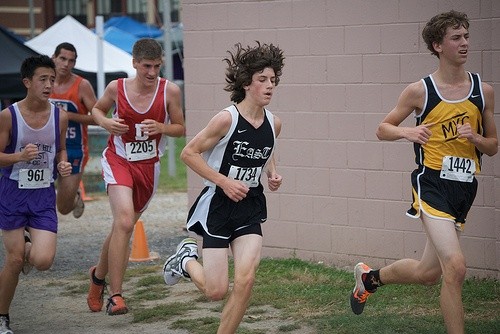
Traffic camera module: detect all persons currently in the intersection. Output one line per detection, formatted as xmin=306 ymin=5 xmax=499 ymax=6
xmin=86 ymin=41 xmax=186 ymax=314
xmin=164 ymin=40 xmax=285 ymax=334
xmin=0 ymin=55 xmax=73 ymax=334
xmin=349 ymin=10 xmax=498 ymax=334
xmin=48 ymin=42 xmax=98 ymax=219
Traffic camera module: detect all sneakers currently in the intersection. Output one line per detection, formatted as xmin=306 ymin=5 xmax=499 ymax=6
xmin=0 ymin=316 xmax=13 ymax=334
xmin=350 ymin=262 xmax=373 ymax=315
xmin=162 ymin=238 xmax=199 ymax=286
xmin=73 ymin=188 xmax=84 ymax=218
xmin=22 ymin=230 xmax=32 ymax=275
xmin=88 ymin=266 xmax=106 ymax=312
xmin=107 ymin=294 xmax=129 ymax=315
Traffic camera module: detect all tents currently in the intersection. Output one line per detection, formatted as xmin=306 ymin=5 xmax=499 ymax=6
xmin=0 ymin=25 xmax=56 ymax=99
xmin=8 ymin=16 xmax=165 ymax=101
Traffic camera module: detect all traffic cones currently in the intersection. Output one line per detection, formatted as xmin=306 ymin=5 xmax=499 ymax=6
xmin=128 ymin=219 xmax=160 ymax=262
